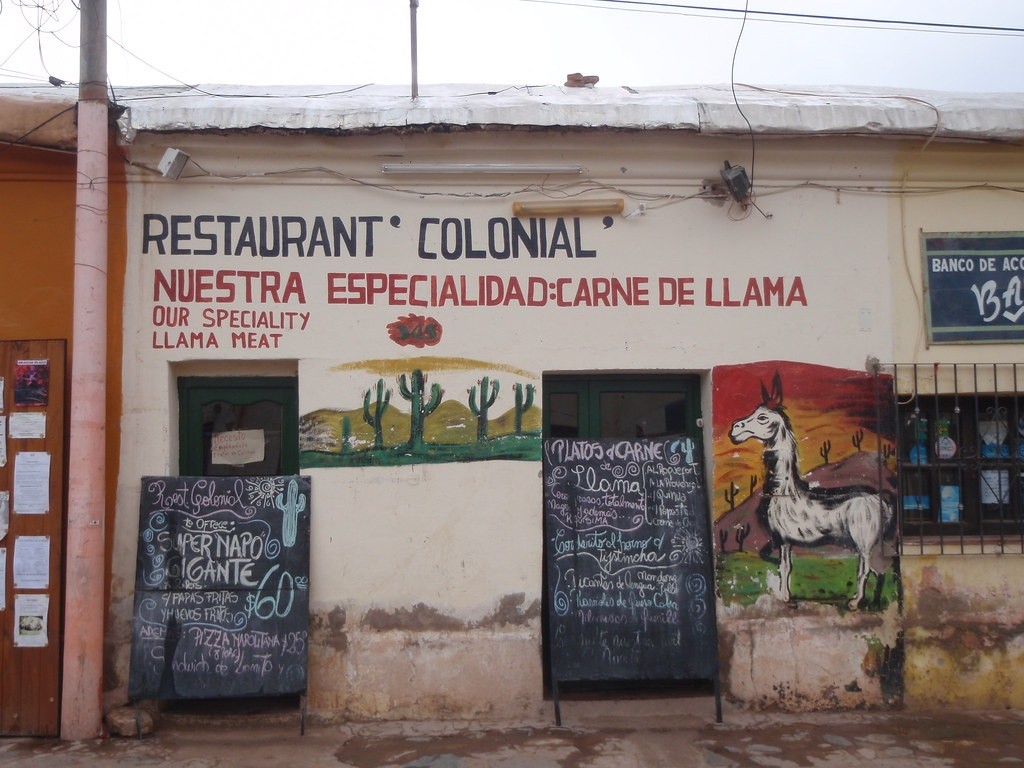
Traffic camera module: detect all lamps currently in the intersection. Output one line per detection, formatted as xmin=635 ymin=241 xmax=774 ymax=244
xmin=379 ymin=162 xmax=581 ymax=175
xmin=512 ymin=198 xmax=625 ymax=217
xmin=155 ymin=144 xmax=190 ymax=181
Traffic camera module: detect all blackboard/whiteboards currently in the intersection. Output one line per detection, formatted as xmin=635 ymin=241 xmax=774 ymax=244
xmin=127 ymin=474 xmax=312 ymax=701
xmin=541 ymin=436 xmax=720 ymax=684
